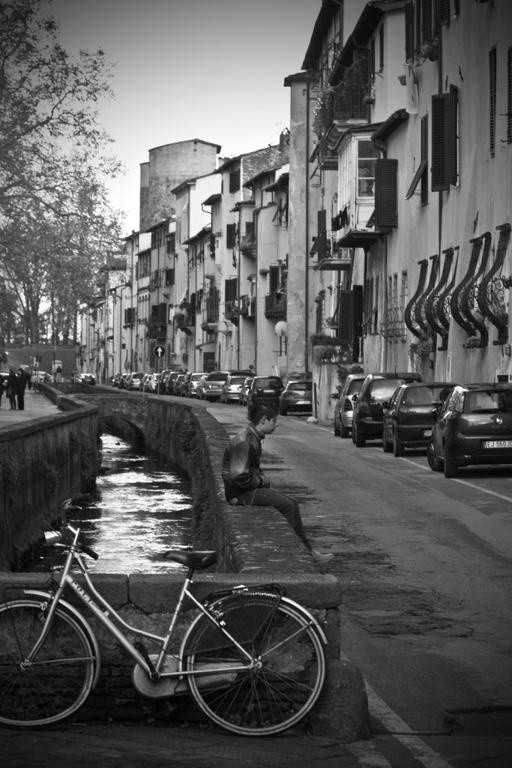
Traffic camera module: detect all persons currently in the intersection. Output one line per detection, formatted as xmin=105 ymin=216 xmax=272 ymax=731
xmin=222 ymin=401 xmax=334 ymax=564
xmin=0 ymin=368 xmax=31 ymax=410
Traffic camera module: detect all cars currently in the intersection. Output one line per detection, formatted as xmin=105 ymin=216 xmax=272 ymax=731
xmin=0 ymin=372 xmax=18 ymax=388
xmin=77 ymin=371 xmax=97 ymax=386
xmin=112 ymin=368 xmax=313 ymax=416
xmin=379 ymin=381 xmax=447 ymax=457
xmin=32 ymin=370 xmax=52 ymax=382
xmin=328 ymin=372 xmax=368 ymax=438
xmin=426 ymin=383 xmax=512 ymax=478
xmin=349 ymin=373 xmax=424 ymax=447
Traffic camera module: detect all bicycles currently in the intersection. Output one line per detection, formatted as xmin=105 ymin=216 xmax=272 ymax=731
xmin=0 ymin=498 xmax=330 ymax=737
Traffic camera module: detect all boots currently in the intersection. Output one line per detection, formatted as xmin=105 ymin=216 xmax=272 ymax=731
xmin=310 ymin=549 xmax=334 ymax=564
